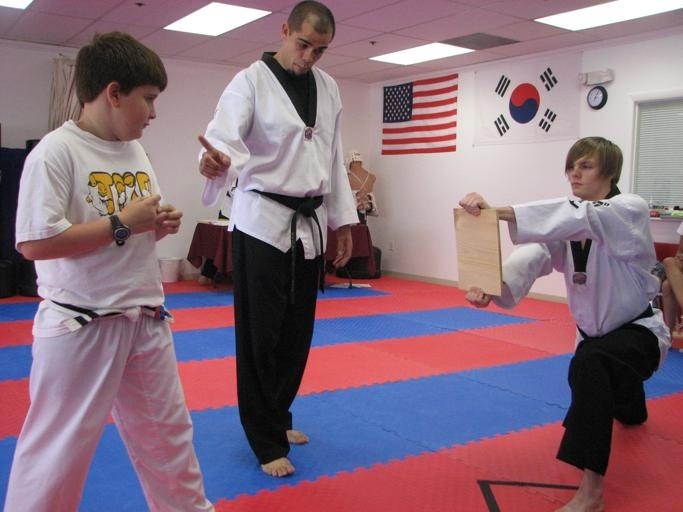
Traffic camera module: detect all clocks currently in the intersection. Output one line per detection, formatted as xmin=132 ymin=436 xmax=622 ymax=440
xmin=586 ymin=86 xmax=607 ymax=110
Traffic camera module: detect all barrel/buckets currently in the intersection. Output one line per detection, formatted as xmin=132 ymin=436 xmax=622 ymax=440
xmin=159 ymin=256 xmax=184 ymax=283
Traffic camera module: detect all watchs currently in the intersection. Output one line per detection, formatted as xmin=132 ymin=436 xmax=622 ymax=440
xmin=109 ymin=214 xmax=132 ymax=248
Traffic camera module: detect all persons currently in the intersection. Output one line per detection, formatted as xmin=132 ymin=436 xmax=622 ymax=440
xmin=458 ymin=137 xmax=671 ymax=511
xmin=661 ymin=219 xmax=683 ymax=332
xmin=198 ymin=1 xmax=360 ymax=478
xmin=344 ymin=151 xmax=376 ymax=211
xmin=3 ymin=32 xmax=216 ymax=512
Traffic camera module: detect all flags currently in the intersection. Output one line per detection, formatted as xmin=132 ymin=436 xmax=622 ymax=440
xmin=381 ymin=74 xmax=458 ymax=154
xmin=474 ymin=50 xmax=583 ymax=145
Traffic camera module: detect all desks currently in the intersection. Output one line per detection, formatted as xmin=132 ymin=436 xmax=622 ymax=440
xmin=197 ymin=222 xmax=369 ymax=289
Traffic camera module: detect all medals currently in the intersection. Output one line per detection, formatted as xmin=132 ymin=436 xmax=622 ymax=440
xmin=572 ymin=271 xmax=588 ymax=285
